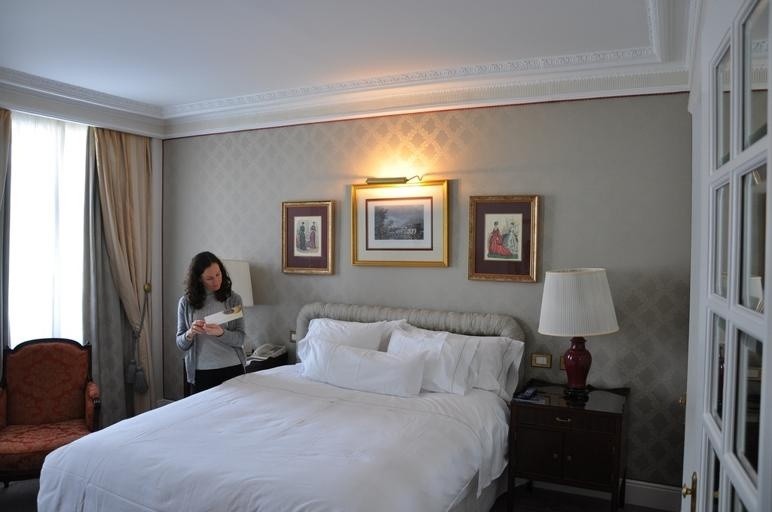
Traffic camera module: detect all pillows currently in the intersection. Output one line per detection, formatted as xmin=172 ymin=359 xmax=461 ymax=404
xmin=296 ymin=319 xmax=525 ymax=398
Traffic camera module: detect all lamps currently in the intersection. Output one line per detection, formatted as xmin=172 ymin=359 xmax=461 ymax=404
xmin=221 ymin=259 xmax=255 ymax=356
xmin=537 ymin=268 xmax=620 ymax=399
xmin=366 ymin=175 xmax=423 ymax=185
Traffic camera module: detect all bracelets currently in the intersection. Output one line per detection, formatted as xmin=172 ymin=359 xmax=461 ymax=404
xmin=187 ymin=334 xmax=192 ymax=339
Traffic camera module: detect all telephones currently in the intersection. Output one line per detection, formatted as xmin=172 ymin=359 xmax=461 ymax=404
xmin=250 ymin=343 xmax=287 ymax=360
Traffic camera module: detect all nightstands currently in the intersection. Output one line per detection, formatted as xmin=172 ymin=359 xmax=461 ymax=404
xmin=507 ymin=379 xmax=628 ymax=511
xmin=182 ymin=348 xmax=288 ymax=398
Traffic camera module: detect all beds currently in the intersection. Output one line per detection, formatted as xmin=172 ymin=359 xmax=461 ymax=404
xmin=37 ymin=301 xmax=532 ymax=511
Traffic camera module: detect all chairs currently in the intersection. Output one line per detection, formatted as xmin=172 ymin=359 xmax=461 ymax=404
xmin=0 ymin=338 xmax=102 ymax=483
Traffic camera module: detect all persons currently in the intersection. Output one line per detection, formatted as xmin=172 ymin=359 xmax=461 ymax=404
xmin=506 ymin=221 xmax=517 ymax=255
xmin=489 ymin=220 xmax=501 ymax=257
xmin=300 ymin=222 xmax=306 ymax=250
xmin=310 ymin=221 xmax=316 ymax=249
xmin=174 ymin=250 xmax=251 ymax=401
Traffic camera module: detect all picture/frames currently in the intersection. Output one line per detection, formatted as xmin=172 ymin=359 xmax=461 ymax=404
xmin=468 ymin=195 xmax=538 ymax=283
xmin=282 ymin=200 xmax=334 ymax=276
xmin=351 ymin=181 xmax=450 ymax=268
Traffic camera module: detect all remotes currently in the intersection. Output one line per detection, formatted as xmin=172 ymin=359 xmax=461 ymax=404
xmin=521 ymin=386 xmax=537 ymax=399
